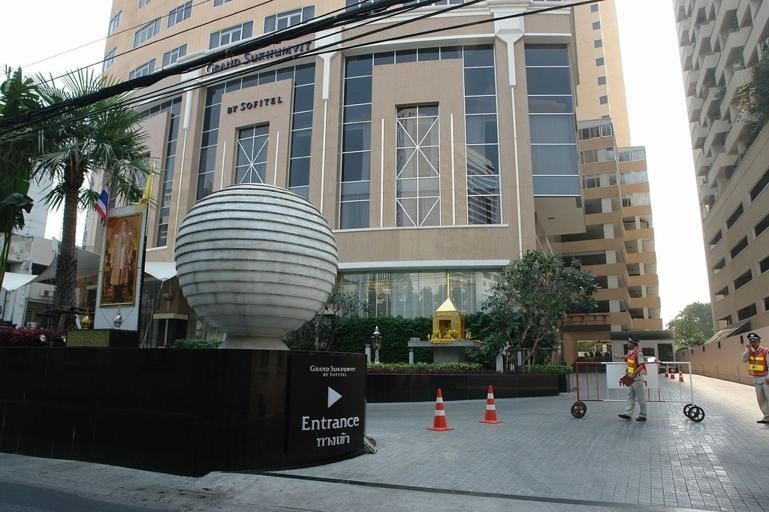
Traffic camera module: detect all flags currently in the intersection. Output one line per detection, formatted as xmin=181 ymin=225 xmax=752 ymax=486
xmin=94 ymin=176 xmax=111 ymax=227
xmin=130 ymin=167 xmax=157 ymax=206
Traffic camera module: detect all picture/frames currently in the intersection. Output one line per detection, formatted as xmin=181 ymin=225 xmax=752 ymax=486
xmin=99 ymin=212 xmax=143 ymax=308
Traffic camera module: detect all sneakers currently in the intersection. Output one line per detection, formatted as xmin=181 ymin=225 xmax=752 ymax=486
xmin=618 ymin=414 xmax=631 ymax=421
xmin=756 ymin=420 xmax=768 ymax=424
xmin=636 ymin=417 xmax=647 ymax=421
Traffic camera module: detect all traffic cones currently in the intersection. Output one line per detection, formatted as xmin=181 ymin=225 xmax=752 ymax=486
xmin=478 ymin=385 xmax=503 ymax=425
xmin=425 ymin=389 xmax=454 ymax=432
xmin=664 ymin=367 xmax=685 ymax=384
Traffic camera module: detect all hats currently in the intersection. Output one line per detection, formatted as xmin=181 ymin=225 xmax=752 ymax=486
xmin=747 ymin=332 xmax=762 ymax=343
xmin=626 ymin=336 xmax=640 ymax=344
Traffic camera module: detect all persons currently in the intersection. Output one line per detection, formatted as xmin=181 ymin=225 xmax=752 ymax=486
xmin=106 ymin=218 xmax=136 ymax=304
xmin=739 ymin=330 xmax=769 ymax=425
xmin=613 ymin=336 xmax=648 ymax=421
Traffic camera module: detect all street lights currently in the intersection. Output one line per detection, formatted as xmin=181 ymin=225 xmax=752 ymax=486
xmin=370 ymin=326 xmax=383 ymax=364
